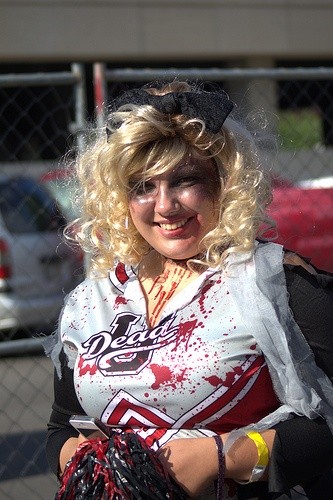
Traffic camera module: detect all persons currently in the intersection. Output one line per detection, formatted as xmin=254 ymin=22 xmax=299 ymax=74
xmin=45 ymin=82 xmax=333 ymax=500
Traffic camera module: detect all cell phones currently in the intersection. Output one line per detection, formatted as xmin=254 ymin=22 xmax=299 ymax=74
xmin=69 ymin=415 xmax=112 ymax=442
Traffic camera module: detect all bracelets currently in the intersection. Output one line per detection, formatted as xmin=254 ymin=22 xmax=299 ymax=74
xmin=234 ymin=429 xmax=268 ymax=484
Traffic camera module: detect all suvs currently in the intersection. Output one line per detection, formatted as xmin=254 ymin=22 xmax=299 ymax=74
xmin=0 ymin=170 xmax=86 ymax=357
